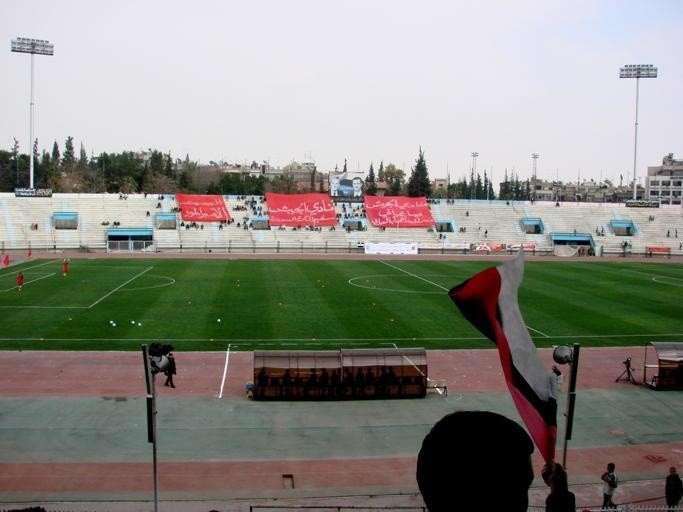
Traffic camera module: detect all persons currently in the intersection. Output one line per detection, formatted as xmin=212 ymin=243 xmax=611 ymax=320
xmin=16 ymin=272 xmax=23 ymax=292
xmin=545 ymin=463 xmax=576 ymax=512
xmin=63 ymin=259 xmax=69 ymax=276
xmin=3 ymin=254 xmax=11 ymax=270
xmin=664 ymin=466 xmax=683 ymax=512
xmin=245 ymin=366 xmax=397 ymax=399
xmin=149 ymin=356 xmax=156 ymax=384
xmin=28 ymin=245 xmax=32 ymax=258
xmin=329 ymin=177 xmax=344 ymax=196
xmin=29 ymin=191 xmax=683 ymax=260
xmin=601 ymin=461 xmax=618 ymax=509
xmin=349 ymin=177 xmax=364 ymax=197
xmin=164 ymin=353 xmax=176 ymax=388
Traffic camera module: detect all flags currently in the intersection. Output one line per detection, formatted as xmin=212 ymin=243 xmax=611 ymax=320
xmin=448 ymin=244 xmax=557 ymax=484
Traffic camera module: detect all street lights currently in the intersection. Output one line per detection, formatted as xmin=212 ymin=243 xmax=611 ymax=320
xmin=620 ymin=65 xmax=657 ymax=200
xmin=12 ymin=38 xmax=54 ymax=189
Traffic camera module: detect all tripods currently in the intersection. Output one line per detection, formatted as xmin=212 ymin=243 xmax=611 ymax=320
xmin=615 ymin=360 xmax=637 ymax=385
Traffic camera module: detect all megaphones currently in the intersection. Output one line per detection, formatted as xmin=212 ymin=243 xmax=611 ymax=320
xmin=150 ymin=354 xmax=171 ymax=374
xmin=553 ymin=343 xmax=573 ymax=365
